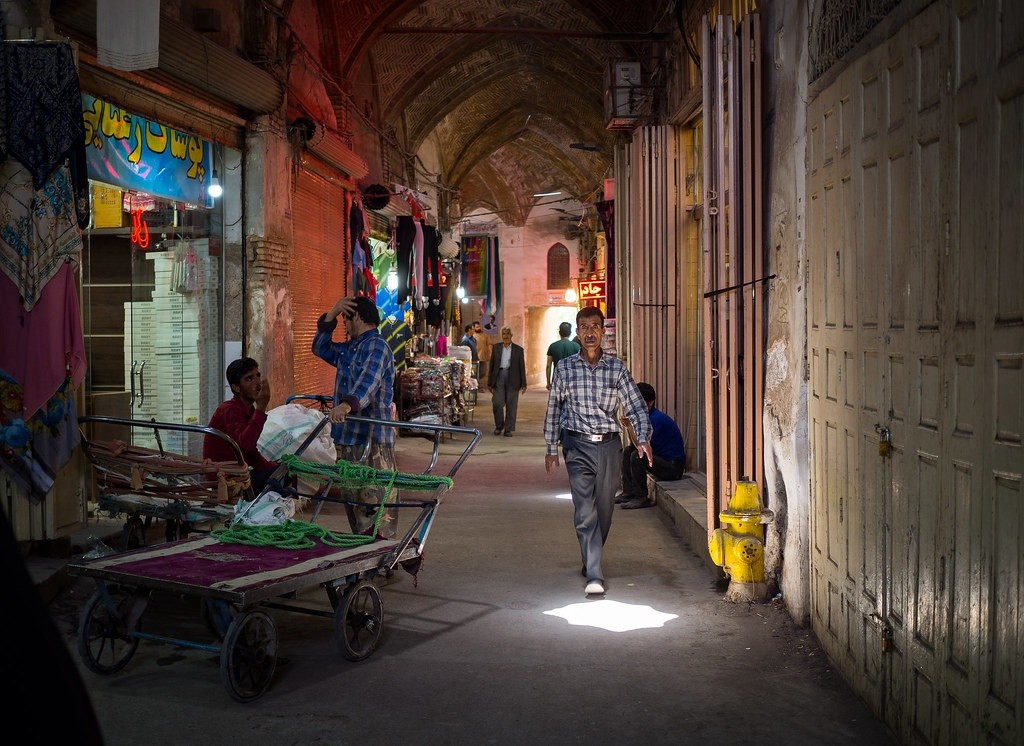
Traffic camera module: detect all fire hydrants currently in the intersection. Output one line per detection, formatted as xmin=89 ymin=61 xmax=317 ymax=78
xmin=708 ymin=477 xmax=775 ymax=605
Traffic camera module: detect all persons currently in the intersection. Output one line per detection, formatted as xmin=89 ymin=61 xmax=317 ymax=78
xmin=202 ymin=358 xmax=297 ymax=499
xmin=472 ymin=321 xmax=491 ymax=393
xmin=614 ymin=382 xmax=685 ymax=509
xmin=544 ymin=307 xmax=653 ymax=595
xmin=485 ymin=315 xmax=496 ymax=330
xmin=490 ymin=326 xmax=528 ymax=438
xmin=461 ymin=325 xmax=479 ymax=373
xmin=545 ymin=322 xmax=580 ymax=390
xmin=312 ymin=297 xmax=398 ymax=590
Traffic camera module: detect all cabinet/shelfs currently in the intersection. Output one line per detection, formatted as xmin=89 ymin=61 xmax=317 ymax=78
xmin=77 ymin=222 xmax=211 ymax=502
xmin=397 ymin=360 xmax=479 ymax=444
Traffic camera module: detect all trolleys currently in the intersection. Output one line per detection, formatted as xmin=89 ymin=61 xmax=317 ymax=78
xmin=63 ymin=395 xmax=483 ymax=705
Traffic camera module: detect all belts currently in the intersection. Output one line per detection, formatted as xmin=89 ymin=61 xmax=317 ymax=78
xmin=566 ymin=429 xmax=617 ymax=442
xmin=499 ymin=368 xmax=510 ymax=370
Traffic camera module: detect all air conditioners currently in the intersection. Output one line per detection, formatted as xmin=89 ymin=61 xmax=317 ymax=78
xmin=601 ymin=57 xmax=641 ymax=127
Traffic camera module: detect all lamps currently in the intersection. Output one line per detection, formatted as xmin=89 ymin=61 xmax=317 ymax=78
xmin=569 ymin=144 xmax=614 ymax=156
xmin=549 ymin=208 xmax=577 ymax=217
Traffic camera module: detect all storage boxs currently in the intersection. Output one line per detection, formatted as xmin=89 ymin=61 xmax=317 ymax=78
xmin=141 ymin=209 xmax=206 ymax=227
xmin=91 ymin=183 xmax=129 ymax=229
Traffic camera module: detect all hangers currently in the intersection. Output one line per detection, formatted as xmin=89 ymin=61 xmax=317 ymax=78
xmin=387 ymin=247 xmax=395 ymax=254
xmin=386 ymin=287 xmax=395 ymax=291
xmin=388 ymin=314 xmax=397 ymax=320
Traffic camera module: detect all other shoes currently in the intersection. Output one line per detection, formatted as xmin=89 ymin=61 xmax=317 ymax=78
xmin=621 ymin=497 xmax=650 ymax=509
xmin=504 ymin=431 xmax=513 ymax=437
xmin=360 ymin=575 xmax=389 ymax=589
xmin=615 ymin=494 xmax=633 ymax=503
xmin=585 ymin=579 xmax=604 ymax=594
xmin=494 ymin=428 xmax=503 ymax=435
